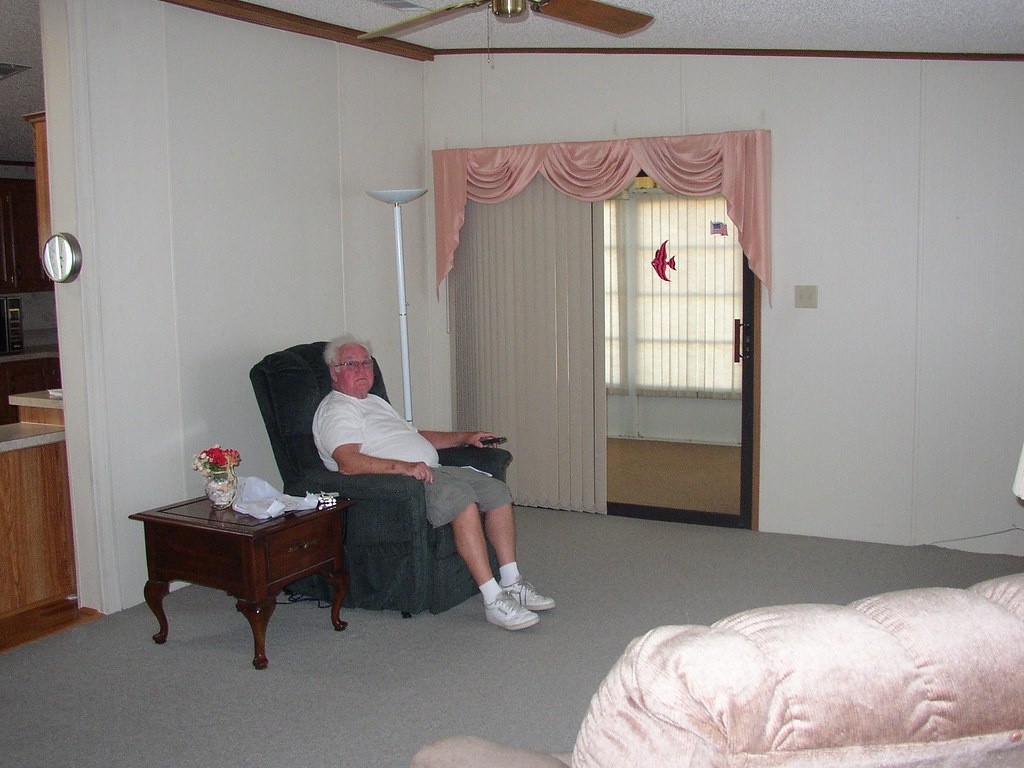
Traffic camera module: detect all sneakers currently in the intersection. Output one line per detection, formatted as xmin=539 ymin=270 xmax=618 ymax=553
xmin=499 ymin=576 xmax=555 ymax=610
xmin=483 ymin=591 xmax=541 ymax=630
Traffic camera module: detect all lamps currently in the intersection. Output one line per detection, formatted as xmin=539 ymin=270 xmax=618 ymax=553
xmin=367 ymin=189 xmax=429 ymax=425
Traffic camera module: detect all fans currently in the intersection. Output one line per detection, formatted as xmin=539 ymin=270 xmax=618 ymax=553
xmin=356 ymin=0 xmax=656 ymax=61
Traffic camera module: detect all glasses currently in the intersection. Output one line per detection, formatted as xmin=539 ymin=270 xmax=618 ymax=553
xmin=333 ymin=359 xmax=372 ymax=370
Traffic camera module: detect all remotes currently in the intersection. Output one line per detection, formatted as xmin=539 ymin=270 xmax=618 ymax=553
xmin=480 ymin=437 xmax=507 ymax=446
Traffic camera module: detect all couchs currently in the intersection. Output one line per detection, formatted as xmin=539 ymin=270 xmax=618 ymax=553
xmin=411 ymin=572 xmax=1024 ymax=768
xmin=250 ymin=340 xmax=513 ymax=618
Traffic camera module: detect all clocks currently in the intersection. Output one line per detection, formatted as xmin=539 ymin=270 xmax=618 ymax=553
xmin=42 ymin=233 xmax=82 ymax=283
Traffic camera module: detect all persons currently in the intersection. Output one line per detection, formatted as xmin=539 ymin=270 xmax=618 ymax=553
xmin=312 ymin=335 xmax=555 ymax=630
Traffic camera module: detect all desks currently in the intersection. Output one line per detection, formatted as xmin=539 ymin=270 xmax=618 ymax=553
xmin=129 ymin=493 xmax=354 ymax=669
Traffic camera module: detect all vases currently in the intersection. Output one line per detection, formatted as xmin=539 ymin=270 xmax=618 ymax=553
xmin=203 ymin=471 xmax=239 ymax=508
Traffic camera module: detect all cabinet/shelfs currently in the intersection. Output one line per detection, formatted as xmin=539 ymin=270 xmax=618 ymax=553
xmin=1 ymin=405 xmax=77 ymax=621
xmin=0 ymin=177 xmax=55 ymax=295
xmin=0 ymin=359 xmax=63 ymax=425
xmin=23 ymin=111 xmax=52 ymax=260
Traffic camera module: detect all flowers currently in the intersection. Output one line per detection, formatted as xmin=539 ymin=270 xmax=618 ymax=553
xmin=192 ymin=445 xmax=241 ymax=477
